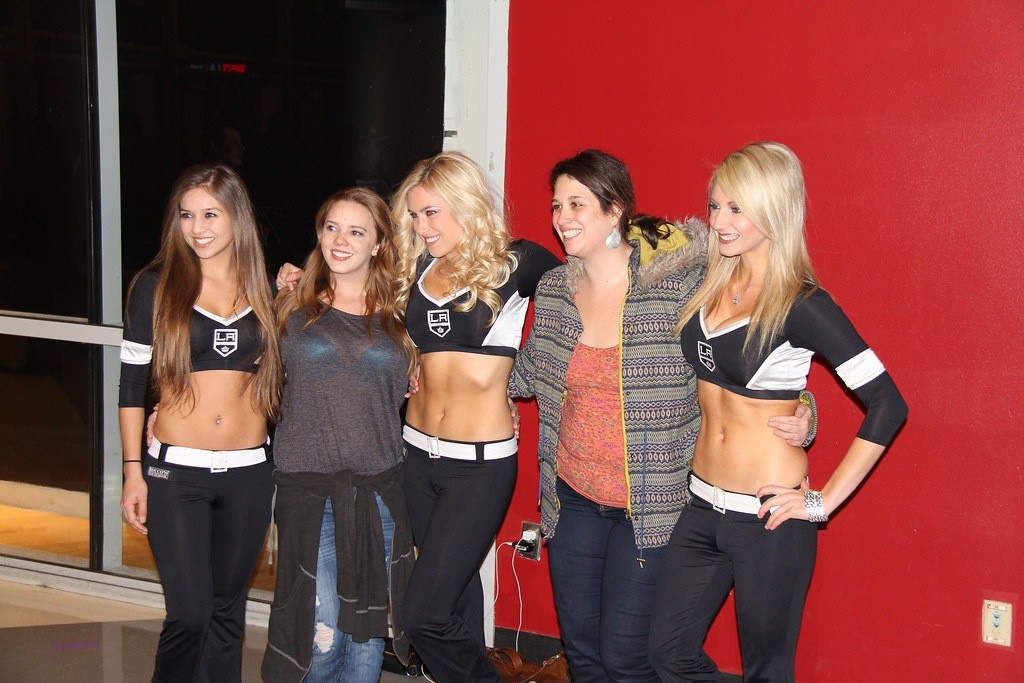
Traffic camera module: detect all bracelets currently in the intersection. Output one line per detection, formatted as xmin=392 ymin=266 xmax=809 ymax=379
xmin=122 ymin=458 xmax=142 ymax=463
xmin=802 ymin=491 xmax=829 ymax=522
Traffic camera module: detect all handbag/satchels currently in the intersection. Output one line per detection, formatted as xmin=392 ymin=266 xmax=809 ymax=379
xmin=381 ymin=637 xmax=430 ymax=677
xmin=487 ymin=646 xmax=572 ymax=683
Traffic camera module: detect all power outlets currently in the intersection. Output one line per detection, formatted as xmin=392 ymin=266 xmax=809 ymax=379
xmin=520 ymin=521 xmax=542 ymax=561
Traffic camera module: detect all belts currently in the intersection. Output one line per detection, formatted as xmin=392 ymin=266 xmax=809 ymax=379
xmin=148 ymin=434 xmax=271 ymax=473
xmin=402 ymin=424 xmax=519 ymax=461
xmin=688 ymin=476 xmax=810 ymax=517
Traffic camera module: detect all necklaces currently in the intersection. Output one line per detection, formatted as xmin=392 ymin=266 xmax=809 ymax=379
xmin=732 ymin=261 xmax=767 ymax=306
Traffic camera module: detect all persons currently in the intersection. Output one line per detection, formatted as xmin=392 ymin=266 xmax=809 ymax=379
xmin=645 ymin=140 xmax=909 ymax=682
xmin=276 ymin=151 xmax=567 ymax=683
xmin=119 ymin=162 xmax=275 ymax=683
xmin=403 ymin=147 xmax=819 ymax=683
xmin=146 ymin=188 xmax=521 ymax=683
